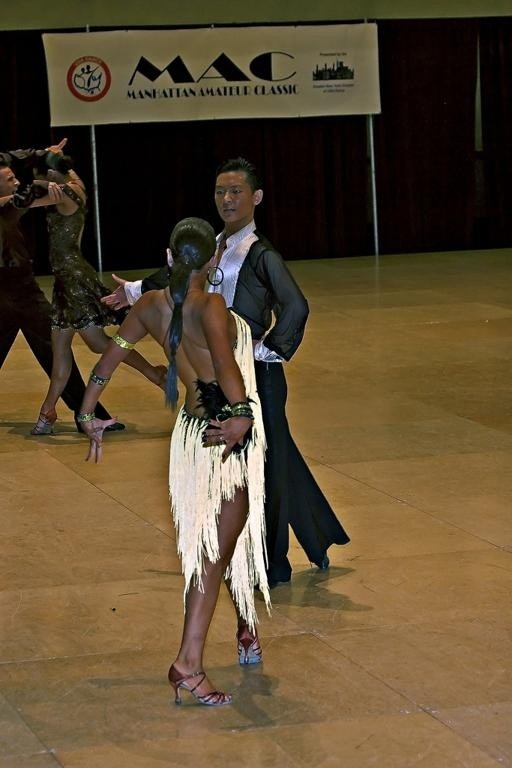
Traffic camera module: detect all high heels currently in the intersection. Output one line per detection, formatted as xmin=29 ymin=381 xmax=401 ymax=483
xmin=236 ymin=623 xmax=263 ymax=665
xmin=30 ymin=410 xmax=57 ymax=435
xmin=168 ymin=664 xmax=233 ymax=705
xmin=156 ymin=365 xmax=179 ymax=404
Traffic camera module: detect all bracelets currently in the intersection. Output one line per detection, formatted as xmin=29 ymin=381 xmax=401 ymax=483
xmin=229 ymin=401 xmax=254 ymax=419
xmin=77 ymin=410 xmax=96 ymax=423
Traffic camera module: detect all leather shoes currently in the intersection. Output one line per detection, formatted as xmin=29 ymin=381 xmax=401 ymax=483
xmin=314 ymin=551 xmax=329 ymax=571
xmin=254 ymin=573 xmax=291 ymax=591
xmin=74 ymin=417 xmax=125 ymax=432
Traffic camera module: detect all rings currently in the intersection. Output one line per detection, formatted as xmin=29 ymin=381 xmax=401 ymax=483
xmin=218 ymin=436 xmax=224 ymax=440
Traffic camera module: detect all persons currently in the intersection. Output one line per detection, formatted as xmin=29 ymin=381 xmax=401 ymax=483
xmin=2 ymin=138 xmax=126 ymax=433
xmin=1 ymin=144 xmax=181 ymax=435
xmin=97 ymin=155 xmax=351 ymax=594
xmin=76 ymin=215 xmax=271 ymax=705
